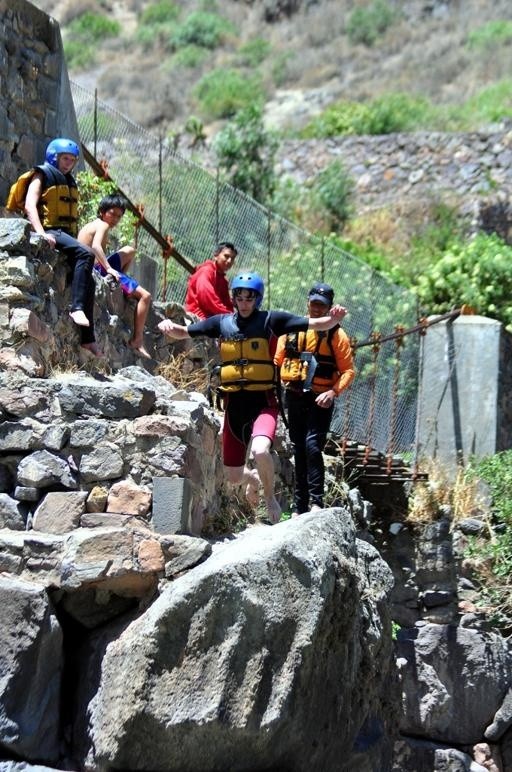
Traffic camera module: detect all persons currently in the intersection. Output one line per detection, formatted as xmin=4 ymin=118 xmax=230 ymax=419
xmin=272 ymin=283 xmax=355 ymax=519
xmin=157 ymin=273 xmax=348 ymax=525
xmin=77 ymin=195 xmax=152 ymax=360
xmin=186 ymin=242 xmax=238 ymax=321
xmin=6 ymin=138 xmax=104 ymax=359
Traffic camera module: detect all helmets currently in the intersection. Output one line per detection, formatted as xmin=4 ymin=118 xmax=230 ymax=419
xmin=231 ymin=273 xmax=264 ymax=310
xmin=46 ymin=138 xmax=80 ymax=169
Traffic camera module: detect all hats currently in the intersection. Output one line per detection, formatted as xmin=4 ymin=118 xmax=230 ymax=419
xmin=308 ymin=283 xmax=333 ymax=306
